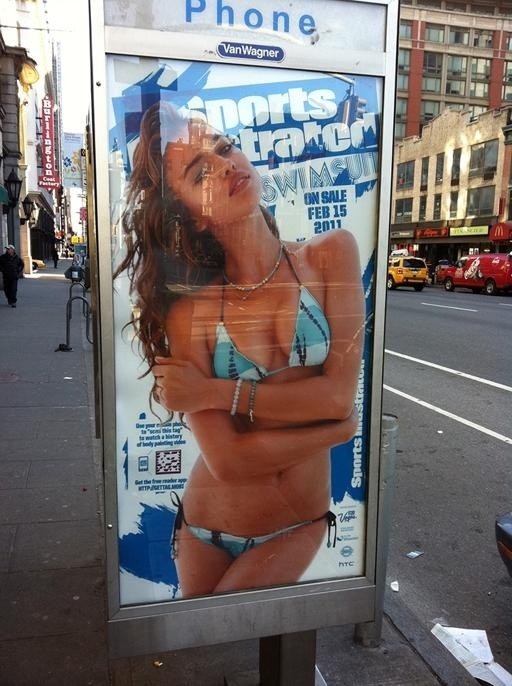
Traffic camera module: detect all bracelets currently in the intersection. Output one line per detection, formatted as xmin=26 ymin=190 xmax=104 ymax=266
xmin=246 ymin=379 xmax=259 ymax=426
xmin=227 ymin=370 xmax=244 ymax=421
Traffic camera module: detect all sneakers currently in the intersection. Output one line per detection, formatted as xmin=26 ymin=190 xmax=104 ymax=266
xmin=11 ymin=303 xmax=16 ymax=308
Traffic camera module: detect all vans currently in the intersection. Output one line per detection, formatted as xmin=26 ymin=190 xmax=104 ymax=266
xmin=436 ymin=254 xmax=511 ymax=295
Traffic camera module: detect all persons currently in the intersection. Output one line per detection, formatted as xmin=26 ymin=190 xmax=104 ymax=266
xmin=1 ymin=243 xmax=25 ymax=308
xmin=113 ymin=95 xmax=372 ymax=601
xmin=49 ymin=247 xmax=61 ymax=269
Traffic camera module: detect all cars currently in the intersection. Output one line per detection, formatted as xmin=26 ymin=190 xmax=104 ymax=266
xmin=387 ymin=257 xmax=427 ymax=292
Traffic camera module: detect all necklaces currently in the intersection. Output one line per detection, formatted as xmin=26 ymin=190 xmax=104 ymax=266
xmin=222 ymin=240 xmax=289 ymax=292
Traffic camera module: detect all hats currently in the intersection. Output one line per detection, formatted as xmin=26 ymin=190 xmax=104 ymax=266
xmin=5 ymin=244 xmax=16 ymax=251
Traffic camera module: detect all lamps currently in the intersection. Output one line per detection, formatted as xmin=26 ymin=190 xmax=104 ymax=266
xmin=20 ymin=194 xmax=34 ymax=225
xmin=3 ymin=167 xmax=22 ymax=215
